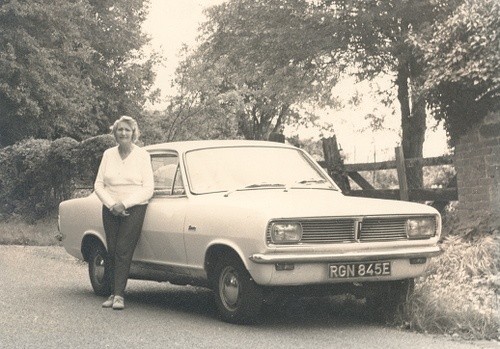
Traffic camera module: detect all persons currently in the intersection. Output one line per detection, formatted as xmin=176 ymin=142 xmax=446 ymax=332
xmin=94 ymin=115 xmax=154 ymax=309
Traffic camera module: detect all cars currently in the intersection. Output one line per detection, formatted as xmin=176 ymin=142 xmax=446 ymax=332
xmin=58 ymin=140 xmax=442 ymax=324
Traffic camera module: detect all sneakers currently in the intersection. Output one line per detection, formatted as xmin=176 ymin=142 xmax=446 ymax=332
xmin=113 ymin=295 xmax=125 ymax=309
xmin=102 ymin=295 xmax=114 ymax=307
